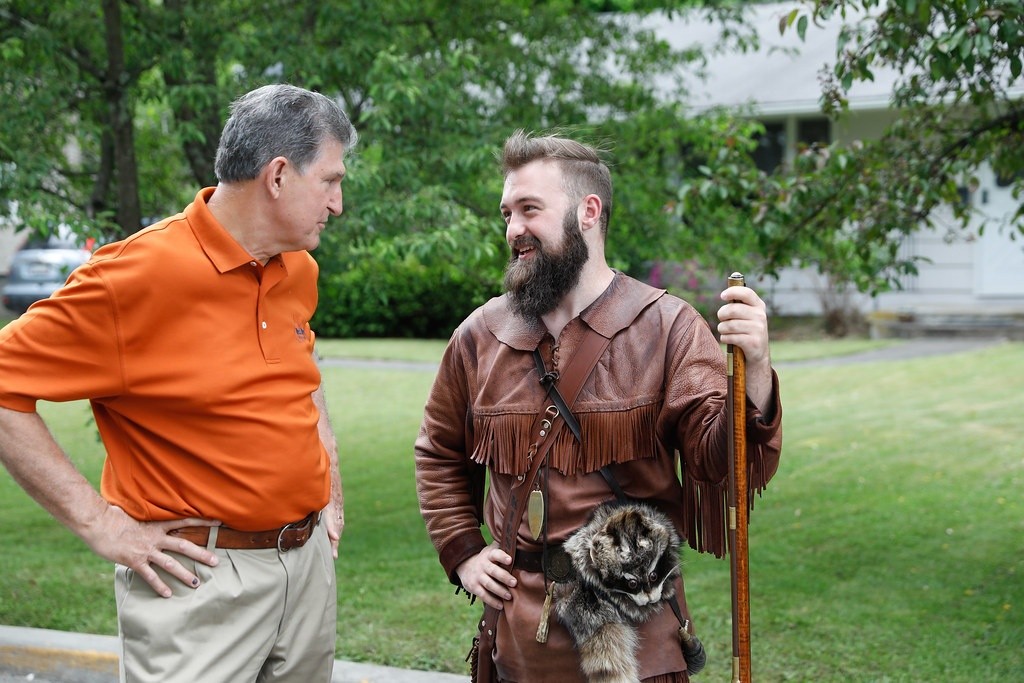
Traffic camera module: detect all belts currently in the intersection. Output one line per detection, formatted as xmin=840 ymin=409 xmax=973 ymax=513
xmin=165 ymin=508 xmax=324 ymax=554
xmin=512 ymin=542 xmax=580 ymax=584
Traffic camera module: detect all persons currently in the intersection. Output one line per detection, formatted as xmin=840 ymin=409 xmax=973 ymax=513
xmin=0 ymin=83 xmax=358 ymax=683
xmin=412 ymin=125 xmax=781 ymax=683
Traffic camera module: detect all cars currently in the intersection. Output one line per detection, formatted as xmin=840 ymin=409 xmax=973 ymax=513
xmin=2 ymin=248 xmax=92 ymax=314
